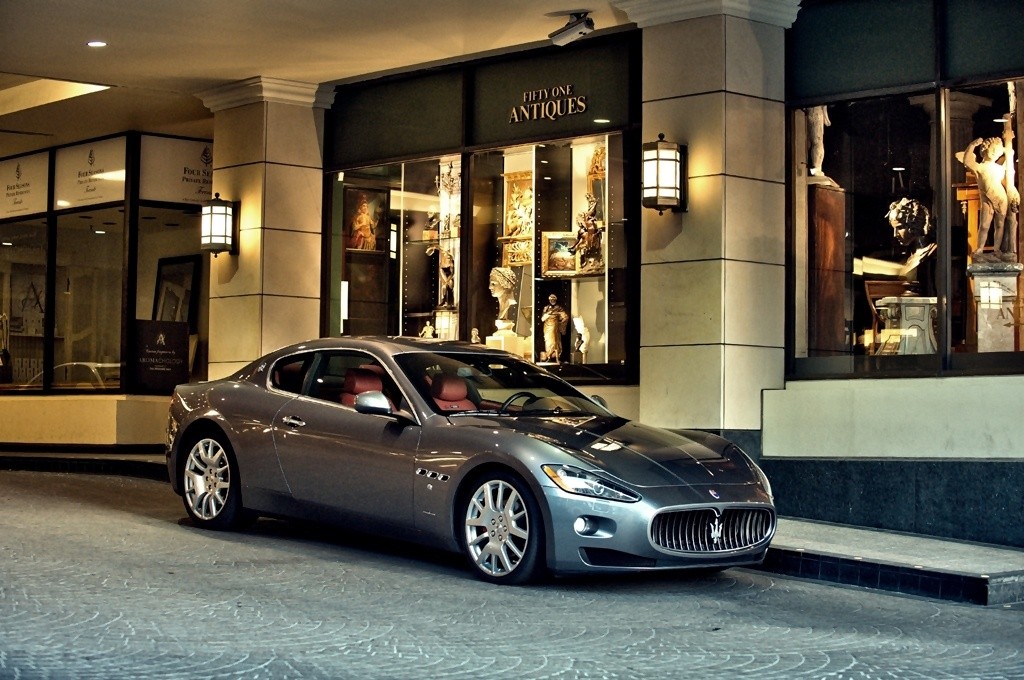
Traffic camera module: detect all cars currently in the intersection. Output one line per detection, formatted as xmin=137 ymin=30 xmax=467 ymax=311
xmin=168 ymin=334 xmax=778 ymax=586
xmin=20 ymin=361 xmax=120 ymax=389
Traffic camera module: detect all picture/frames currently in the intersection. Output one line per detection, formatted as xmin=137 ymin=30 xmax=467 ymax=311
xmin=541 ymin=231 xmax=581 ymax=276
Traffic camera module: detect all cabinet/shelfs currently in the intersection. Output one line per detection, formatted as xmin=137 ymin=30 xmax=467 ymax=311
xmin=439 ymin=131 xmax=637 ymax=376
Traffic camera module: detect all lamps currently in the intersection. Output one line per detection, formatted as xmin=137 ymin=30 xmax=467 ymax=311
xmin=201 ymin=193 xmax=240 ymax=257
xmin=642 ymin=133 xmax=689 ymax=215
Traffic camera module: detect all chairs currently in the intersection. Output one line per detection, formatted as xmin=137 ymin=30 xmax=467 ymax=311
xmin=431 ymin=373 xmax=476 ymax=411
xmin=275 ymin=363 xmax=305 ymax=393
xmin=359 ymin=364 xmax=392 ymax=397
xmin=342 ymin=368 xmax=395 ymax=413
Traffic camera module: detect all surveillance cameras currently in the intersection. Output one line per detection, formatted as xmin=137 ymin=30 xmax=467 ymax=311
xmin=548 ymin=17 xmax=594 ymax=47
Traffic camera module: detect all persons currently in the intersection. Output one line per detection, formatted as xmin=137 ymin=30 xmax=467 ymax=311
xmin=885 ymin=198 xmax=937 ymax=282
xmin=488 ymin=266 xmax=518 ymax=320
xmin=426 ymin=246 xmax=454 ymax=306
xmin=964 ymin=136 xmax=1008 ymax=262
xmin=566 ymin=198 xmax=605 ymax=269
xmin=470 ymin=328 xmax=481 ymax=343
xmin=806 ymin=105 xmax=831 ymax=177
xmin=540 ymin=293 xmax=569 ymax=363
xmin=419 ymin=321 xmax=435 ymax=339
